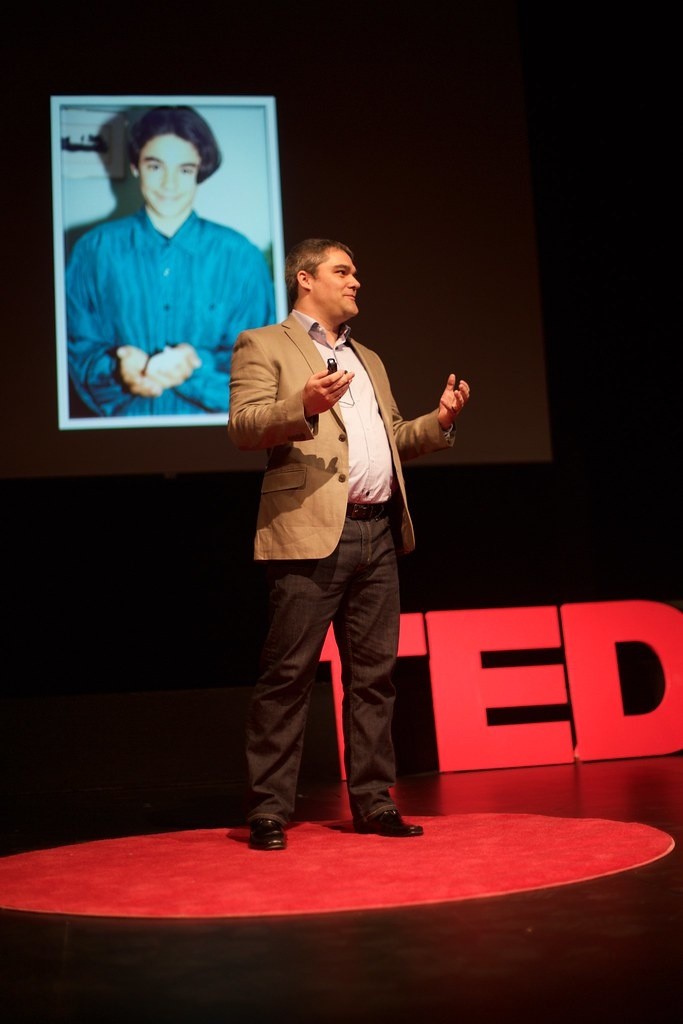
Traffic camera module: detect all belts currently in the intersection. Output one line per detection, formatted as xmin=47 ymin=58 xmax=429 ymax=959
xmin=346 ymin=502 xmax=396 ymax=519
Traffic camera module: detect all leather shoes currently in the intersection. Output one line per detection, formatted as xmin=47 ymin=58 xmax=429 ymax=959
xmin=249 ymin=819 xmax=284 ymax=849
xmin=354 ymin=809 xmax=423 ymax=837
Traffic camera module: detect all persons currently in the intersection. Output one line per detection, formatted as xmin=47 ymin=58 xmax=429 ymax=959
xmin=65 ymin=104 xmax=276 ymax=421
xmin=228 ymin=240 xmax=471 ymax=851
